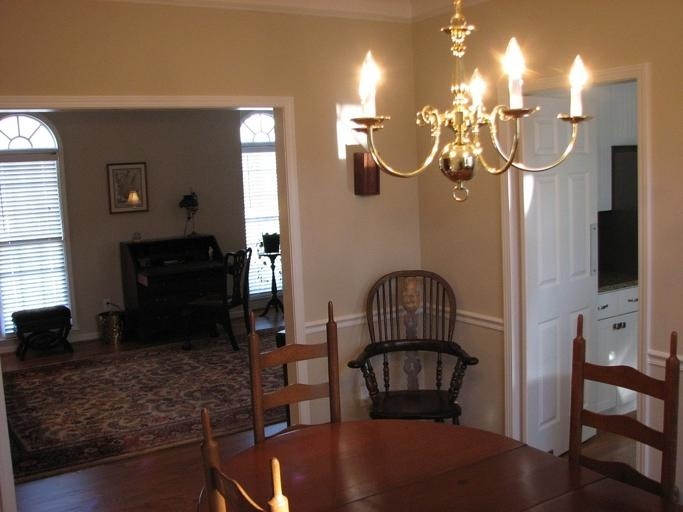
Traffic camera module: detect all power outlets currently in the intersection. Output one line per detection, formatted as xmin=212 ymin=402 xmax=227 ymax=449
xmin=104 ymin=298 xmax=112 ymax=312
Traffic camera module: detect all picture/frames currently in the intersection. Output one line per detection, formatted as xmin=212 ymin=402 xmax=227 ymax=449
xmin=106 ymin=162 xmax=150 ymax=214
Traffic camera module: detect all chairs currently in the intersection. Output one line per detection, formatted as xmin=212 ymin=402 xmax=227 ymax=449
xmin=200 ymin=408 xmax=288 ymax=512
xmin=345 ymin=269 xmax=478 ymax=427
xmin=192 ymin=248 xmax=251 ymax=350
xmin=248 ymin=301 xmax=341 ymax=441
xmin=567 ymin=312 xmax=682 ymax=498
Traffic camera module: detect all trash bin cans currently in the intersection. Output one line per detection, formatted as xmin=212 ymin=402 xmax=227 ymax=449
xmin=96 ymin=310 xmax=124 ymax=345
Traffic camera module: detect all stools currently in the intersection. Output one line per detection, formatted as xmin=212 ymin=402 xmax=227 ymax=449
xmin=11 ymin=307 xmax=72 ymax=359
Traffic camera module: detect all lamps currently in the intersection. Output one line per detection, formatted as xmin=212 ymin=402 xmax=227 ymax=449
xmin=344 ymin=0 xmax=592 ymax=204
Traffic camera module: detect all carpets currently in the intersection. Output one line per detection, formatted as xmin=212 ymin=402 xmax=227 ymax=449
xmin=0 ymin=326 xmax=288 ymax=484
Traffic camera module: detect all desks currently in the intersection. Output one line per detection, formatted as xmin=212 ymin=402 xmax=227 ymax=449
xmin=121 ymin=232 xmax=226 ymax=343
xmin=258 ymin=252 xmax=285 ymax=317
xmin=216 ymin=419 xmax=670 ymax=511
